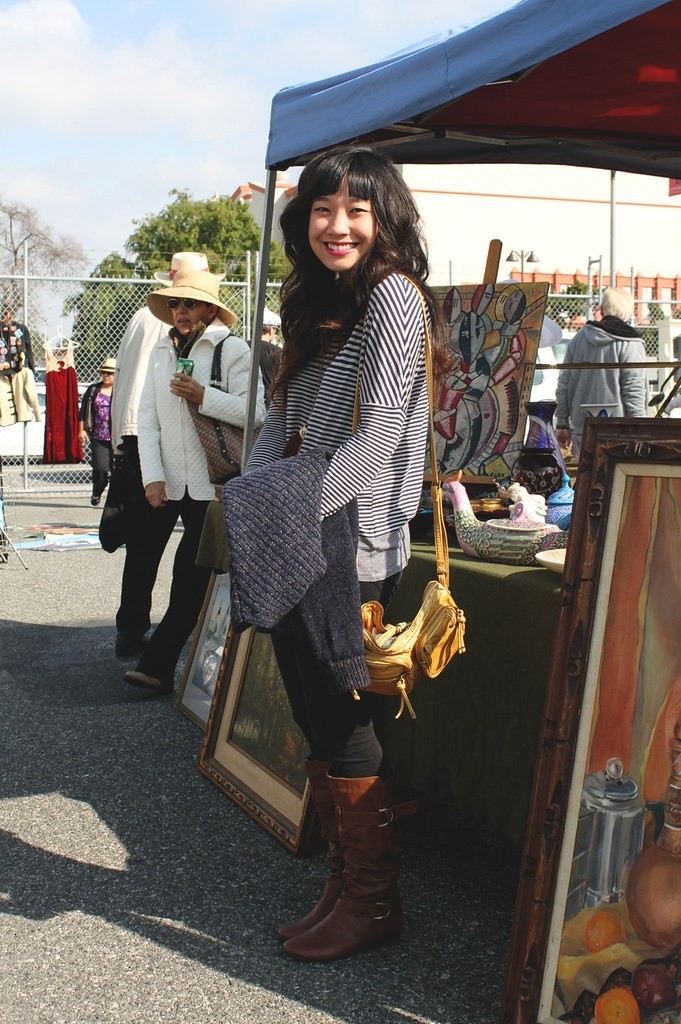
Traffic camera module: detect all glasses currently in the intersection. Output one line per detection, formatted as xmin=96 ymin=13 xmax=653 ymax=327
xmin=166 ymin=298 xmax=195 ymax=311
xmin=101 ymin=371 xmax=113 ymax=375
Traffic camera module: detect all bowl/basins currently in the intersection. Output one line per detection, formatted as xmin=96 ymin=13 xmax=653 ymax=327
xmin=469 ymin=498 xmax=502 ymax=512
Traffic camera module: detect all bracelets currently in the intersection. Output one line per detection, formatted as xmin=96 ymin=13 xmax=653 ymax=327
xmin=556 ymin=425 xmax=569 ymax=430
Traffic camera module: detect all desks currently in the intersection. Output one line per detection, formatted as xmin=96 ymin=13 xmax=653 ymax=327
xmin=193 ymin=500 xmax=567 ymax=858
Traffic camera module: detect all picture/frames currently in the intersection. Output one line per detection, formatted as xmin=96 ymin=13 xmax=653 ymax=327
xmin=194 ymin=596 xmax=317 ymax=855
xmin=173 ymin=568 xmax=238 ymax=729
xmin=499 ymin=415 xmax=681 ymax=1024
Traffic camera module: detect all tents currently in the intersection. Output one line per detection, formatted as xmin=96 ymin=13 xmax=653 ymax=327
xmin=241 ymin=0 xmax=681 ymax=473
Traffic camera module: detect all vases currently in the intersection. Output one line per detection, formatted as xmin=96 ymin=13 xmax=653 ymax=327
xmin=580 ymin=404 xmax=620 ymax=416
xmin=513 ymin=446 xmax=564 ymax=497
xmin=523 ymin=401 xmax=570 ymax=489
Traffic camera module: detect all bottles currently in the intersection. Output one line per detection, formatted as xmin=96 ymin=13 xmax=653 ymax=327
xmin=580 ymin=403 xmax=620 ymax=418
xmin=511 ymin=447 xmax=563 ymax=501
xmin=545 ymin=473 xmax=575 ymax=531
xmin=523 ymin=401 xmax=565 ymax=470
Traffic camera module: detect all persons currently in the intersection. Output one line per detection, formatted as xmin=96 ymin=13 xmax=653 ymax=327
xmin=556 ymin=288 xmax=647 ymax=455
xmin=247 ymin=311 xmax=283 ymax=395
xmin=78 ymin=358 xmax=115 ymax=505
xmin=123 ymin=271 xmax=267 ymax=692
xmin=223 ymin=145 xmax=454 ymax=963
xmin=112 ymin=251 xmax=209 ymax=658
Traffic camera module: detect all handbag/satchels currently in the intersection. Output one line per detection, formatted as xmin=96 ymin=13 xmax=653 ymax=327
xmin=349 ymin=580 xmax=467 ymax=721
xmin=184 ymin=331 xmax=264 ymax=484
xmin=532 ymin=355 xmax=543 ymax=385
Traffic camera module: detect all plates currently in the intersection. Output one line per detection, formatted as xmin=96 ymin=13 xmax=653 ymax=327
xmin=534 ymin=548 xmax=566 ymax=574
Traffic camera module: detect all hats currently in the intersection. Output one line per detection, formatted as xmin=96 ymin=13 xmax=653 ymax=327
xmin=97 ymin=358 xmax=116 ymax=372
xmin=154 ymin=252 xmax=226 ymax=287
xmin=147 ymin=269 xmax=237 ymax=327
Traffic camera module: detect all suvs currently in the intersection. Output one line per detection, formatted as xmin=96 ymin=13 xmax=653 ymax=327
xmin=529 ymin=332 xmax=577 ymax=402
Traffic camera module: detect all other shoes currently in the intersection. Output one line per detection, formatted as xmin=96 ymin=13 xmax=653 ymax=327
xmin=123 ymin=658 xmax=175 ymax=693
xmin=115 ymin=632 xmax=149 ymax=658
xmin=91 ymin=493 xmax=100 ymax=506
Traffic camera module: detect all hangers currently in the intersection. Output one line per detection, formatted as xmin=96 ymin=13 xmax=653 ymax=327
xmin=42 ymin=324 xmax=80 ymax=351
xmin=1 ymin=309 xmax=14 ymax=325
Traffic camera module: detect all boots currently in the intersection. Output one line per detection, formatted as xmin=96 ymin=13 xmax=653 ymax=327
xmin=275 ymin=757 xmax=419 ymax=961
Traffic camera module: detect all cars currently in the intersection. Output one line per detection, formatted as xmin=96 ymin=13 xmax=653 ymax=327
xmin=0 ymin=367 xmax=88 ymax=462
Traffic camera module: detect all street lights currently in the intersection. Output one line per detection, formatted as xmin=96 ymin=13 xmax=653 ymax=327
xmin=506 ymin=250 xmax=539 ymax=284
